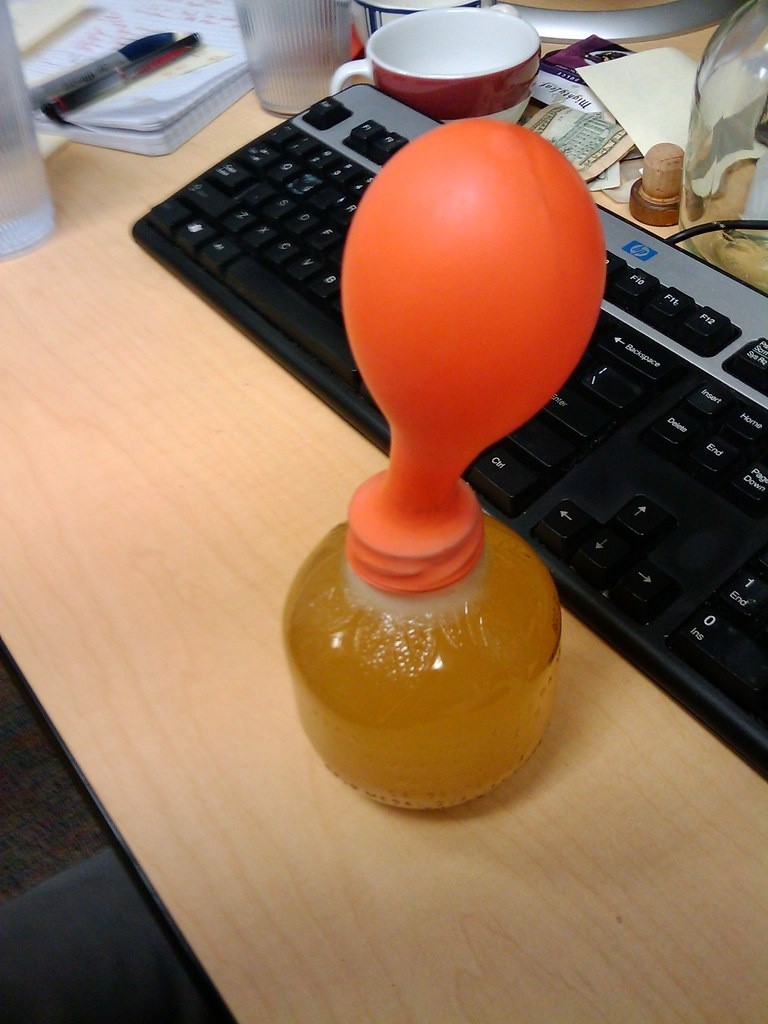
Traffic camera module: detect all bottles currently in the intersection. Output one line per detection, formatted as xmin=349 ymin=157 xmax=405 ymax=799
xmin=679 ymin=0 xmax=767 ymax=296
xmin=281 ymin=467 xmax=564 ymax=809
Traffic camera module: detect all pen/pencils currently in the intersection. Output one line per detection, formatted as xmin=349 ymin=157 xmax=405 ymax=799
xmin=41 ymin=31 xmax=201 ymax=119
xmin=26 ymin=32 xmax=174 ymax=110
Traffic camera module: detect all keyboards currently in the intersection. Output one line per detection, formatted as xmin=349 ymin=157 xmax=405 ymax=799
xmin=131 ymin=85 xmax=767 ymax=784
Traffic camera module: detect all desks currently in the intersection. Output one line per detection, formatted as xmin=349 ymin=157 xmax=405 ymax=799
xmin=0 ymin=0 xmax=768 ymax=1024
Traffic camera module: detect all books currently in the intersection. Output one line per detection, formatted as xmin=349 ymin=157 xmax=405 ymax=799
xmin=12 ymin=0 xmax=255 ymax=157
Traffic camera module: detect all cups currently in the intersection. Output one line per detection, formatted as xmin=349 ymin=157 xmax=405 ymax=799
xmin=234 ymin=0 xmax=352 ymax=121
xmin=0 ymin=0 xmax=58 ymax=262
xmin=351 ymin=0 xmax=521 ymax=47
xmin=330 ymin=7 xmax=541 ymax=124
